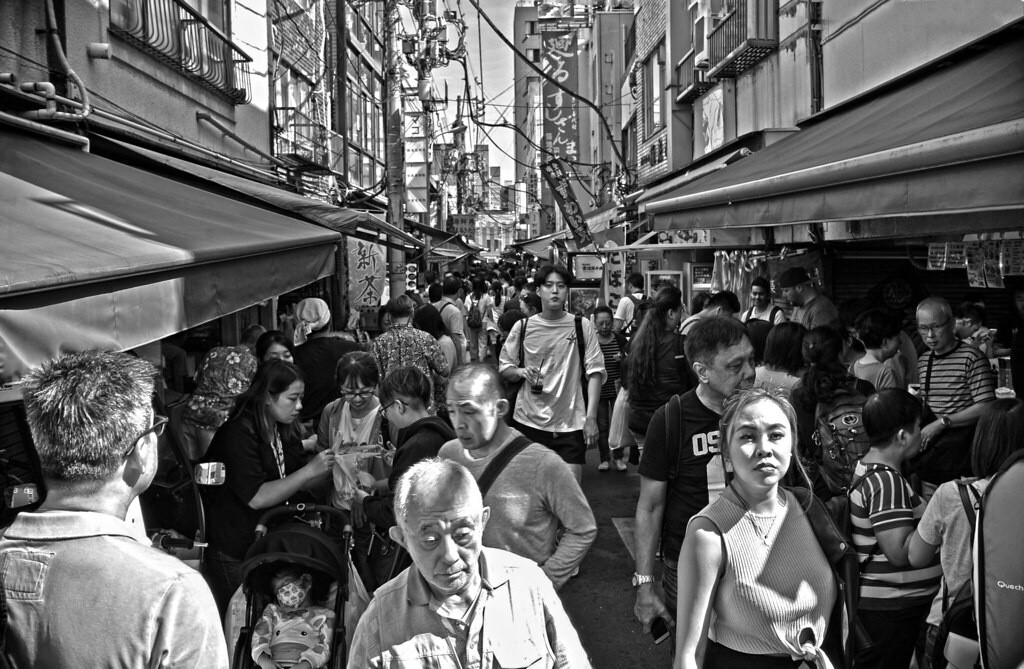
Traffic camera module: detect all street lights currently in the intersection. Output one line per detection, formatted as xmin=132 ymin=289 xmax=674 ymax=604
xmin=423 ymin=125 xmax=469 ymax=269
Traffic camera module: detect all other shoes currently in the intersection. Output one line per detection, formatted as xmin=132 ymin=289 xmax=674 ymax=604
xmin=571 ymin=566 xmax=580 ymax=578
xmin=612 ymin=457 xmax=627 ymax=471
xmin=598 ymin=460 xmax=609 ymax=471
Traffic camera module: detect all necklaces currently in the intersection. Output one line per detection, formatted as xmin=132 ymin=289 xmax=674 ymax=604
xmin=747 ymin=497 xmax=780 ymax=546
xmin=350 ymin=399 xmax=375 ymax=431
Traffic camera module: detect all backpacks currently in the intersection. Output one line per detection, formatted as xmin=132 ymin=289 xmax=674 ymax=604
xmin=932 ymin=484 xmax=977 ymax=669
xmin=808 ymin=374 xmax=872 ymax=496
xmin=621 ymin=293 xmax=648 ymax=337
xmin=824 ymin=468 xmax=905 ymax=598
xmin=466 ymin=294 xmax=486 ymax=329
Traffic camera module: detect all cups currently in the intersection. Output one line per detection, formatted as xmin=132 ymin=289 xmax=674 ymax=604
xmin=530 ymin=372 xmax=544 ymax=394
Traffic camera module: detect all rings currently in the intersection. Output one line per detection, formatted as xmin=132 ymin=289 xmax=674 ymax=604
xmin=325 ymin=450 xmax=328 ymax=454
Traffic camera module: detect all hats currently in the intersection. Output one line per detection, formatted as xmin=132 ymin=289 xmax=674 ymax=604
xmin=779 ymin=266 xmax=818 ymax=288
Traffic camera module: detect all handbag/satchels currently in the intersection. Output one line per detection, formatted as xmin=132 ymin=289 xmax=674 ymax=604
xmin=505 ymin=318 xmax=529 ymax=427
xmin=943 ymin=631 xmax=982 ymax=669
xmin=606 ymin=386 xmax=635 ymax=450
xmin=349 ymin=524 xmax=412 ymax=593
xmin=326 ymin=434 xmax=386 ymax=511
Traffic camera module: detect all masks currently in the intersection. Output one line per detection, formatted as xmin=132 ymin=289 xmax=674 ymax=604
xmin=276 ymin=576 xmax=311 ymax=608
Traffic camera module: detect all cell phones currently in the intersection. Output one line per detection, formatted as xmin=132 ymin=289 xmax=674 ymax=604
xmin=651 ymin=616 xmax=671 ymax=645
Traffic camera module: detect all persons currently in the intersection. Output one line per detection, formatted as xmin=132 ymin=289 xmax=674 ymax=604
xmin=251 ymin=568 xmax=336 ymax=669
xmin=439 ymin=367 xmax=597 ymax=588
xmin=347 ymin=457 xmax=591 ymax=669
xmin=139 ymin=251 xmax=1023 ymax=669
xmin=674 ymin=387 xmax=849 ymax=669
xmin=632 ymin=317 xmax=756 ymax=632
xmin=0 ymin=350 xmax=230 ymax=669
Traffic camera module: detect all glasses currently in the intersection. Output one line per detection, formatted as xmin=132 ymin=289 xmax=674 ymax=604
xmin=124 ymin=415 xmax=169 ymax=457
xmin=338 ymin=382 xmax=377 ymax=399
xmin=378 ymin=400 xmax=408 ymax=418
xmin=917 ymin=319 xmax=951 ymax=334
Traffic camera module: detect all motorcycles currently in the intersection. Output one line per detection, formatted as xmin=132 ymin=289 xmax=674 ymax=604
xmin=0 ymin=395 xmax=227 ymax=575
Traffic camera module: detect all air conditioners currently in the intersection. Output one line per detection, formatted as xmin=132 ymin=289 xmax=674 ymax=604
xmin=692 ymin=11 xmax=726 ymax=66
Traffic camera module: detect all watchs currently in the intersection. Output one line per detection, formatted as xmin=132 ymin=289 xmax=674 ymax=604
xmin=632 ymin=572 xmax=655 ymax=590
xmin=941 ymin=416 xmax=952 ymax=429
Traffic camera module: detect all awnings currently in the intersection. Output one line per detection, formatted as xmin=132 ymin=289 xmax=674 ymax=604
xmin=645 ymin=36 xmax=1024 ymax=231
xmin=89 ymin=128 xmax=424 ymax=255
xmin=0 ymin=112 xmax=343 ymax=402
xmin=362 ymin=199 xmax=480 ymax=266
xmin=511 ymin=229 xmax=566 ymax=255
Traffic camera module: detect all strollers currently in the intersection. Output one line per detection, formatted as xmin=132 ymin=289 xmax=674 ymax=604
xmin=231 ymin=501 xmax=353 ymax=669
